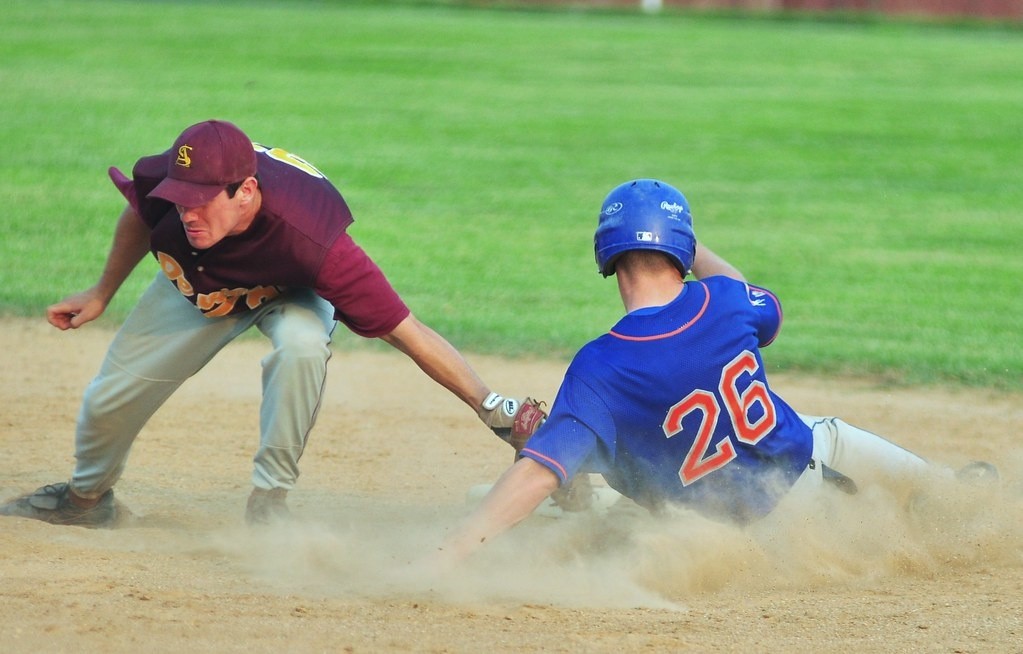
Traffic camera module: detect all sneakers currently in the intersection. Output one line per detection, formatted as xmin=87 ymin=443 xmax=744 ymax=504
xmin=0 ymin=482 xmax=117 ymax=529
xmin=246 ymin=485 xmax=291 ymax=527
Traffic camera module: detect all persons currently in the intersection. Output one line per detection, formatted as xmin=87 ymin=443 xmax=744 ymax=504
xmin=444 ymin=178 xmax=1002 ymax=545
xmin=0 ymin=119 xmax=548 ymax=528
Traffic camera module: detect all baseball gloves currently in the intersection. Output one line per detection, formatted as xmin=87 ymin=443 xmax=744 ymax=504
xmin=476 ymin=388 xmax=606 ymax=517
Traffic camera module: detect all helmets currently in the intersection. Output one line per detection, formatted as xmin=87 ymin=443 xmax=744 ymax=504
xmin=593 ymin=181 xmax=696 ymax=275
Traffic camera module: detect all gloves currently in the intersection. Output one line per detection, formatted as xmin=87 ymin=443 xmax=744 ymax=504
xmin=479 ymin=389 xmax=518 ymax=439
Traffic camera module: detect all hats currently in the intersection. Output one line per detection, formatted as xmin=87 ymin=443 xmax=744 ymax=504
xmin=147 ymin=119 xmax=258 ymax=208
